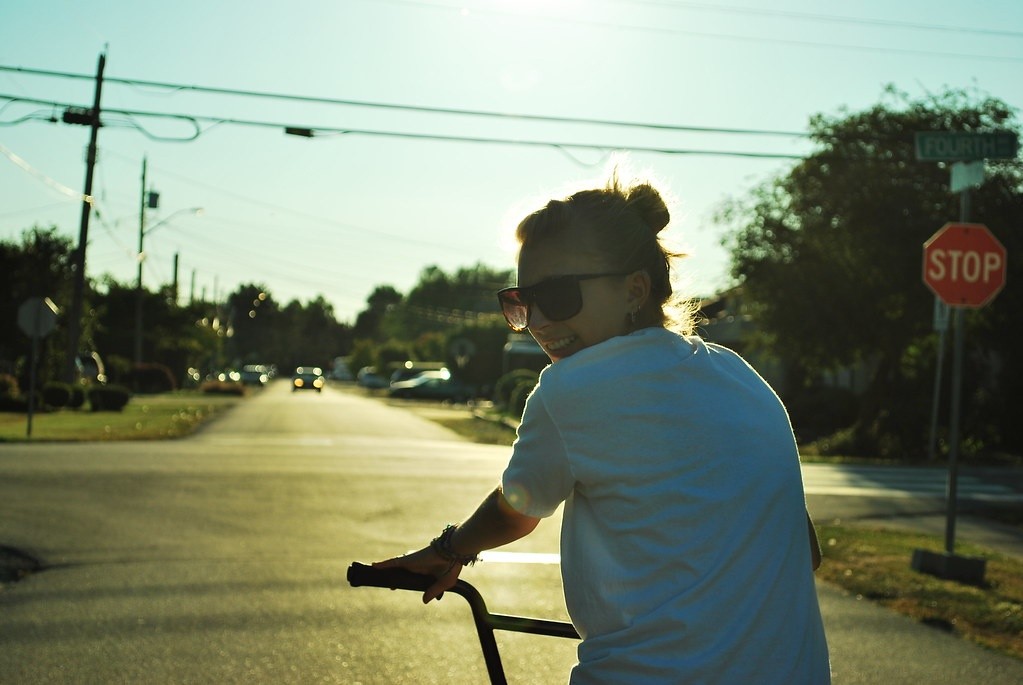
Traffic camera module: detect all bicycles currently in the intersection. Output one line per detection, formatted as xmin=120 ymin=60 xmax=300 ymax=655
xmin=346 ymin=559 xmax=581 ymax=685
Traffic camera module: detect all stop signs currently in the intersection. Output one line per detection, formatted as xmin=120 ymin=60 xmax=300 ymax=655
xmin=921 ymin=221 xmax=1008 ymax=308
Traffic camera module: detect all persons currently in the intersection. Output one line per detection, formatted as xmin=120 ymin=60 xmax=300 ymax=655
xmin=373 ymin=165 xmax=833 ymax=685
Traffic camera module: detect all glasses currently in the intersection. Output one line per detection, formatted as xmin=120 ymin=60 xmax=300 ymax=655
xmin=496 ymin=270 xmax=636 ymax=332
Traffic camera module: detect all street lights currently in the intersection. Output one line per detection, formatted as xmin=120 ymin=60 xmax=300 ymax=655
xmin=135 ymin=207 xmax=204 ymax=393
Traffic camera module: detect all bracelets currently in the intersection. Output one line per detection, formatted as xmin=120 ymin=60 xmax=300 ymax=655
xmin=431 ymin=525 xmax=479 ymax=566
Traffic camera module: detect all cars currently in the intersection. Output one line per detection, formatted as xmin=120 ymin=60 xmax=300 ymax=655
xmin=390 ymin=371 xmax=444 ymax=395
xmin=292 ymin=366 xmax=321 ymax=393
xmin=241 ymin=365 xmax=265 ymax=387
xmin=357 ymin=366 xmax=388 ymax=387
